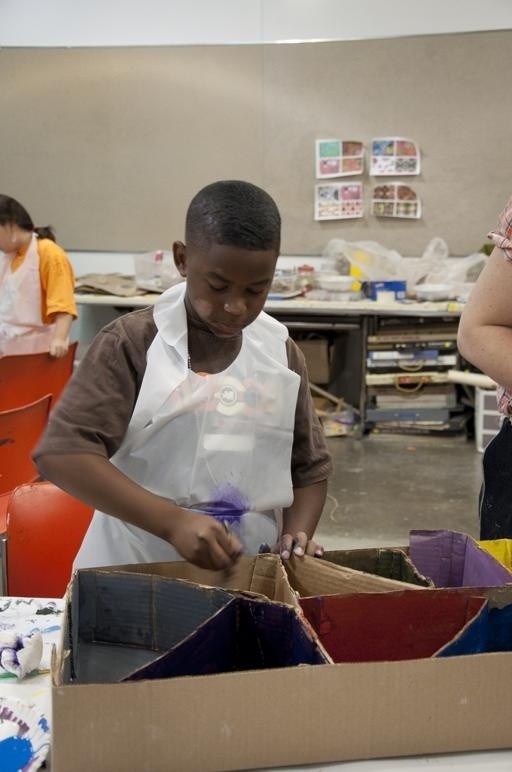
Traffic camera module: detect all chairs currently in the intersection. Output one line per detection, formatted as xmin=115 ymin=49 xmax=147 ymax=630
xmin=1 ymin=343 xmax=76 ymax=409
xmin=1 ymin=482 xmax=96 ymax=599
xmin=0 ymin=394 xmax=53 ymax=533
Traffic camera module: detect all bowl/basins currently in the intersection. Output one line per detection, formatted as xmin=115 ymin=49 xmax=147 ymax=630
xmin=415 ymin=283 xmax=455 ymax=302
xmin=317 ymin=276 xmax=356 ymax=292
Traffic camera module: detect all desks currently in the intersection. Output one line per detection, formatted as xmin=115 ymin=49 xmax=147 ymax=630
xmin=1 ymin=594 xmax=511 ymax=770
xmin=66 ymin=250 xmax=462 ymax=438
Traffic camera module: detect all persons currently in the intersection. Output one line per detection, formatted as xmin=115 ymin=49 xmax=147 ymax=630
xmin=457 ymin=194 xmax=512 ymax=541
xmin=0 ymin=193 xmax=78 ymax=358
xmin=30 ymin=180 xmax=334 ymax=602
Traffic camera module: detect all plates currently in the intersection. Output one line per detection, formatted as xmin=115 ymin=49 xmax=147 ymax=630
xmin=267 ymin=286 xmax=306 ymax=301
